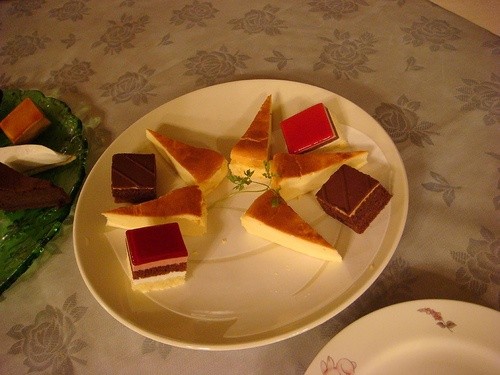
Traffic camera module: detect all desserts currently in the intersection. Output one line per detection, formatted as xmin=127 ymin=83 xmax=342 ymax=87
xmin=270 ymin=148 xmax=370 ymax=201
xmin=239 ymin=188 xmax=343 ymax=265
xmin=0 ymin=97 xmax=52 ymax=145
xmin=125 ymin=222 xmax=190 ymax=292
xmin=315 ymin=164 xmax=394 ymax=235
xmin=100 ymin=185 xmax=208 ymax=238
xmin=110 ymin=152 xmax=158 ymax=207
xmin=0 ymin=163 xmax=73 ymax=211
xmin=228 ymin=93 xmax=273 ymax=186
xmin=0 ymin=142 xmax=77 ymax=176
xmin=146 ymin=127 xmax=230 ymax=198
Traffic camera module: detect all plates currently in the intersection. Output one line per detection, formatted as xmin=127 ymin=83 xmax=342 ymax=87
xmin=0 ymin=86 xmax=88 ymax=297
xmin=303 ymin=299 xmax=500 ymax=375
xmin=72 ymin=77 xmax=409 ymax=349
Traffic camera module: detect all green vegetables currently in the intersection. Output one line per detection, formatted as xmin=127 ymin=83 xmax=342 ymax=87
xmin=225 ymin=159 xmax=288 ymax=208
